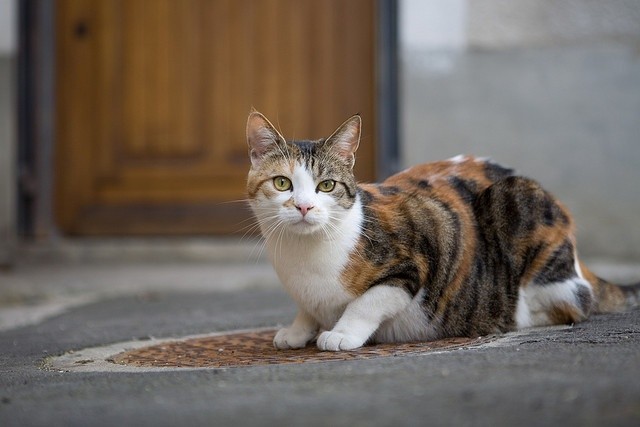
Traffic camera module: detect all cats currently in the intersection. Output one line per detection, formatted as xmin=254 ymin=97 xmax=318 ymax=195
xmin=245 ymin=111 xmax=640 ymax=352
